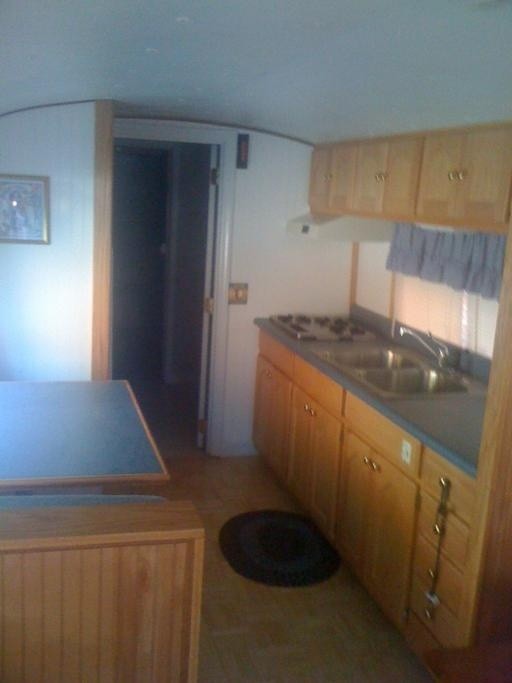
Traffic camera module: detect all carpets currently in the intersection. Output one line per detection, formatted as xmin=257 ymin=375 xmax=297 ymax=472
xmin=220 ymin=511 xmax=340 ymax=587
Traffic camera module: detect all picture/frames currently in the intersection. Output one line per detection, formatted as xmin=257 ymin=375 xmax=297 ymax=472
xmin=0 ymin=175 xmax=50 ymax=244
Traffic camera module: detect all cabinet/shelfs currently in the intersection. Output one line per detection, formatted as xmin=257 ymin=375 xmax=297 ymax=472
xmin=309 ymin=123 xmax=512 ymax=235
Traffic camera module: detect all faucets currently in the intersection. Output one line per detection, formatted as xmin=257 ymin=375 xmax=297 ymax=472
xmin=398 ymin=325 xmax=450 ymax=366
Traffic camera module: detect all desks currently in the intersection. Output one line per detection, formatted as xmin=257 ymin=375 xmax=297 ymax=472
xmin=0 ymin=380 xmax=170 ymax=495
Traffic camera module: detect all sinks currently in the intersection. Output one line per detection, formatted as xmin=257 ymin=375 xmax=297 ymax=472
xmin=356 ymin=366 xmax=473 ymax=397
xmin=321 ymin=345 xmax=420 ymax=372
